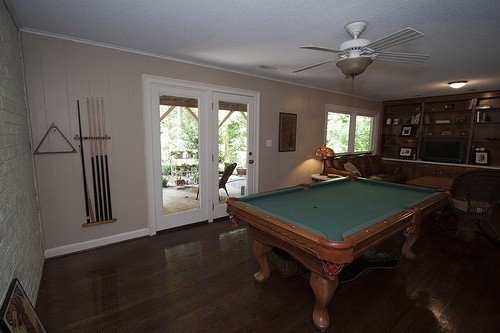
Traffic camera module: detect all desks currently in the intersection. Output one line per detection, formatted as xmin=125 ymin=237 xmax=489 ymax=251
xmin=226 ymin=176 xmax=448 ymax=332
xmin=408 ymin=174 xmax=454 ymax=216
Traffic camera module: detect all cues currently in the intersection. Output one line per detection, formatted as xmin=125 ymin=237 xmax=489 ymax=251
xmin=77 ymin=96 xmax=112 ymax=223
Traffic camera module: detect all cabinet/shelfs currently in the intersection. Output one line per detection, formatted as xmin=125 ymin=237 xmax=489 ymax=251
xmin=379 ymin=90 xmax=500 ymax=166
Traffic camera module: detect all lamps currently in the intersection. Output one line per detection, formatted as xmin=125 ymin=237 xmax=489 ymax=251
xmin=315 ymin=145 xmax=335 ymax=176
xmin=335 ymin=57 xmax=373 ymax=79
xmin=448 ymin=80 xmax=468 ymax=89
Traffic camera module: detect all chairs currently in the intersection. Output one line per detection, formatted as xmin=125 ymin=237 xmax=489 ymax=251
xmin=195 ymin=162 xmax=237 ymax=203
xmin=436 ymin=169 xmax=500 ymax=243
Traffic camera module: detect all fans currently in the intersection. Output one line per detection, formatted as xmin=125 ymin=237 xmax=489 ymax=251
xmin=290 ymin=22 xmax=430 ymax=74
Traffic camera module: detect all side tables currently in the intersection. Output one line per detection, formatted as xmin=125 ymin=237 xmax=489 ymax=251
xmin=310 ymin=173 xmax=342 ymax=183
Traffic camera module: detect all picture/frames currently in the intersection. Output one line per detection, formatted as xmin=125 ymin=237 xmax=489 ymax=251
xmin=0 ymin=278 xmax=48 ymax=333
xmin=400 ymin=124 xmax=413 ymax=137
xmin=473 ymin=151 xmax=491 ymax=166
xmin=278 ymin=112 xmax=297 ymax=152
xmin=399 ymin=146 xmax=413 ymax=158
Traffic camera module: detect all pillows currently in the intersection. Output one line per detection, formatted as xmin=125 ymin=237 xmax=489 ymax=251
xmin=333 ymin=157 xmax=346 ymax=170
xmin=368 ymin=154 xmax=383 ymax=175
xmin=358 ymin=155 xmax=372 ymax=178
xmin=348 ymin=156 xmax=360 ymax=171
xmin=344 ymin=162 xmax=361 ymax=177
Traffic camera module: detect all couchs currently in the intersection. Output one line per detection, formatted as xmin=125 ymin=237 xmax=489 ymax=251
xmin=325 ymin=152 xmax=405 ymax=183
